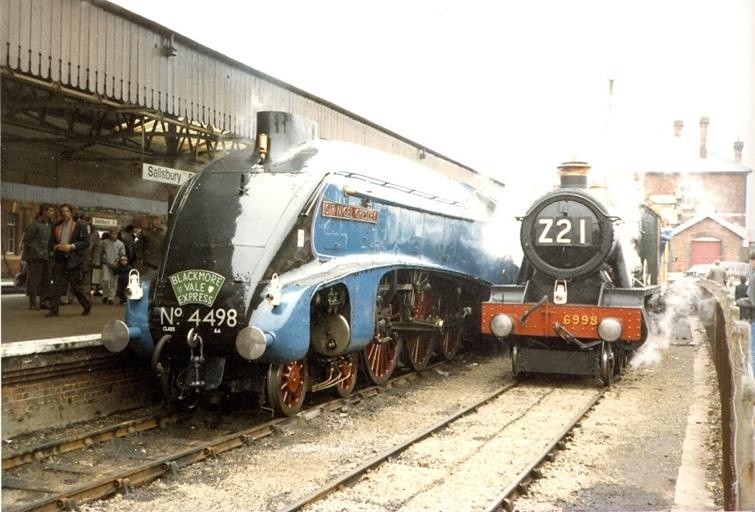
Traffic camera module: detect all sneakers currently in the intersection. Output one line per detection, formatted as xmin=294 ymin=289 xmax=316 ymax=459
xmin=31 ymin=301 xmax=91 ymax=317
xmin=103 ymin=297 xmax=127 ymax=305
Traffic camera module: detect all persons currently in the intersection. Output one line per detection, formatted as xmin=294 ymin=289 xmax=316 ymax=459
xmin=734 ymin=250 xmax=755 ymax=377
xmin=45 ymin=203 xmax=93 ymax=318
xmin=61 ymin=213 xmax=170 ymax=306
xmin=20 ymin=202 xmax=56 ymax=311
xmin=705 ymin=259 xmax=729 ymax=288
xmin=735 ymin=275 xmax=749 ymax=321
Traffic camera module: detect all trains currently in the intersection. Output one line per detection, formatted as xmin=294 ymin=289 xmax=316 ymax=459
xmin=476 ymin=160 xmax=671 ymax=387
xmin=101 ymin=110 xmax=518 ymax=417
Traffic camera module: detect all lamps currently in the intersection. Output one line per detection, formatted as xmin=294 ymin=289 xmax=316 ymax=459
xmin=165 ymin=31 xmax=178 ymax=58
